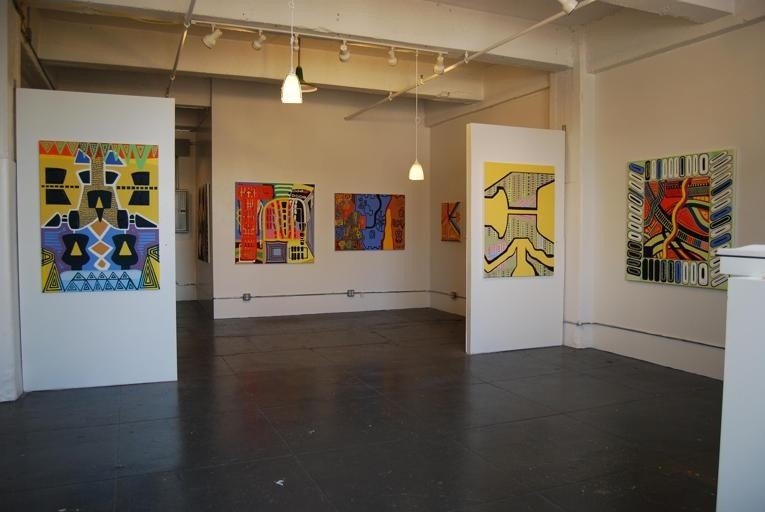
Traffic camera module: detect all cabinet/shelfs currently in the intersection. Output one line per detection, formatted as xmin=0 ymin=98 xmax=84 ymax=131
xmin=715 ymin=244 xmax=765 ymax=512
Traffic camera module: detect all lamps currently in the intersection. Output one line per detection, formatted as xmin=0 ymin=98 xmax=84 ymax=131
xmin=189 ymin=20 xmax=448 ymax=75
xmin=280 ymin=0 xmax=303 ymax=103
xmin=293 ymin=36 xmax=320 ymax=97
xmin=557 ymin=0 xmax=579 ymax=16
xmin=408 ymin=51 xmax=424 ymax=181
